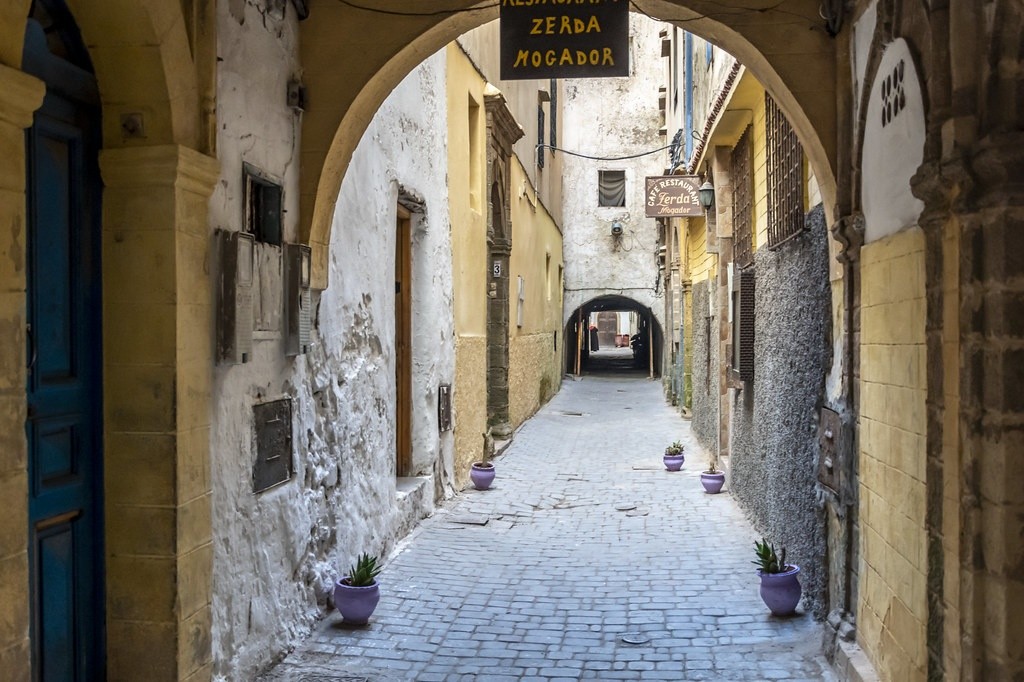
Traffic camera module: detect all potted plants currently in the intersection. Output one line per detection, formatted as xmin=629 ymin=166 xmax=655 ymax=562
xmin=335 ymin=550 xmax=379 ymax=626
xmin=470 ymin=427 xmax=496 ymax=491
xmin=663 ymin=439 xmax=684 ymax=471
xmin=699 ymin=461 xmax=725 ymax=494
xmin=751 ymin=536 xmax=802 ymax=616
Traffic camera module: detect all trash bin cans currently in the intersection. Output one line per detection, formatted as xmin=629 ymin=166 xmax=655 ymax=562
xmin=623 ymin=334 xmax=629 ymax=347
xmin=614 ymin=335 xmax=623 ymax=347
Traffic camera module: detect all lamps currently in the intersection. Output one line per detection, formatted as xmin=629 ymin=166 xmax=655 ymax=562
xmin=698 ymin=161 xmax=714 ymax=209
